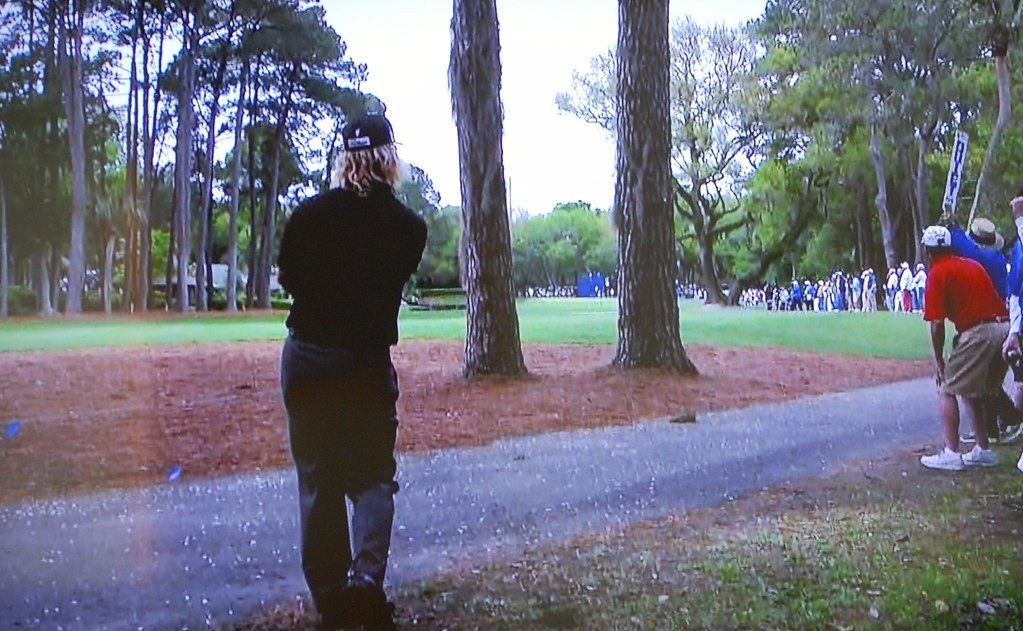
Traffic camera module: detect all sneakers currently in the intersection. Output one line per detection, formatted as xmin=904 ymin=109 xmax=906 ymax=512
xmin=962 ymin=447 xmax=999 ymax=466
xmin=999 ymin=421 xmax=1023 ymax=442
xmin=920 ymin=449 xmax=967 ymax=470
xmin=960 ymin=430 xmax=997 ymax=443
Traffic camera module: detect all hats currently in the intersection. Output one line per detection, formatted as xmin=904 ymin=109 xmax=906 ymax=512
xmin=965 ymin=218 xmax=1003 ymax=250
xmin=342 ymin=116 xmax=396 ymax=152
xmin=920 ymin=226 xmax=951 ymax=246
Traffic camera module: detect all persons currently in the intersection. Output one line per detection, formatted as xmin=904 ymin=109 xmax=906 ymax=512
xmin=1001 ymin=192 xmax=1023 ymax=474
xmin=516 ymin=261 xmax=927 ymax=312
xmin=275 ymin=115 xmax=428 ymax=631
xmin=946 ymin=216 xmax=1022 ymax=444
xmin=920 ymin=224 xmax=1011 ymax=470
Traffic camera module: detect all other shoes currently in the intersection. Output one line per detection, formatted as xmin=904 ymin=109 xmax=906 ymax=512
xmin=320 ymin=598 xmax=393 ymax=631
xmin=346 ymin=580 xmax=399 ymax=631
